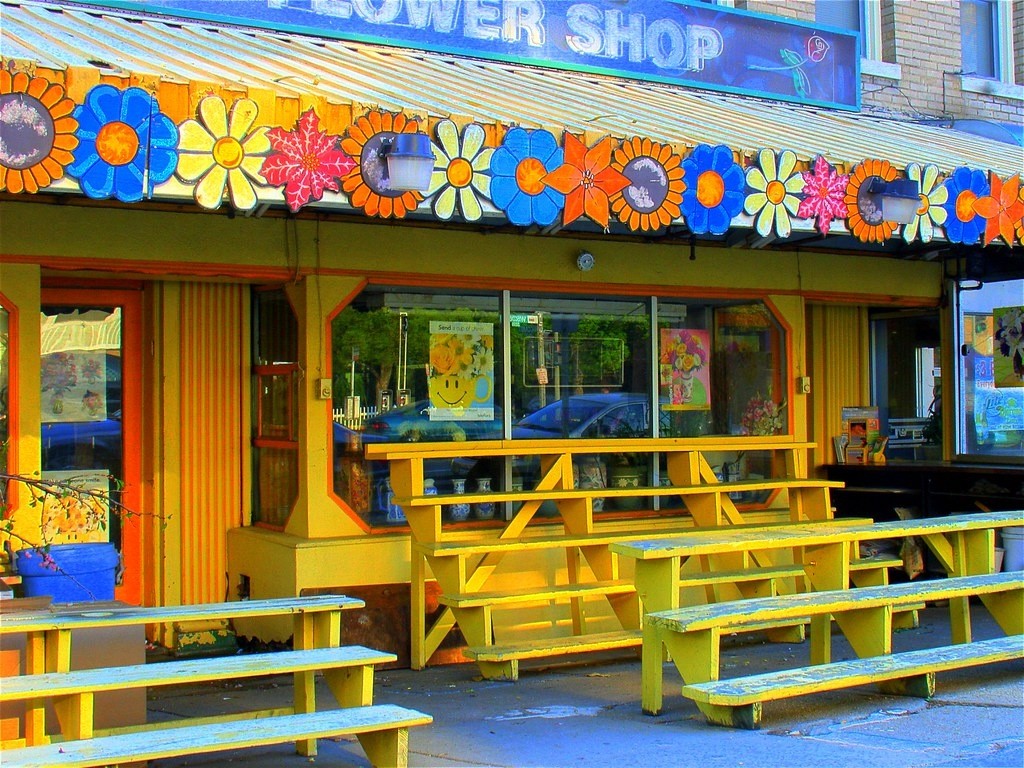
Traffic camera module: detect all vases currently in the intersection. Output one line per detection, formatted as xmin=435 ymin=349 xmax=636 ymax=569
xmin=376 ymin=476 xmax=408 ymax=524
xmin=650 ymin=471 xmax=684 ymax=510
xmin=610 ymin=464 xmax=649 ymax=511
xmin=449 ymin=478 xmax=473 ymax=523
xmin=728 ymin=455 xmax=747 ymax=502
xmin=579 ymin=455 xmax=608 ymax=513
xmin=475 ymin=477 xmax=495 ymax=522
xmin=713 ymin=464 xmax=725 ymax=484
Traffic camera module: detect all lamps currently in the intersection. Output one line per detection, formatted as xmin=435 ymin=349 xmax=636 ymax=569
xmin=856 ymin=175 xmax=925 ymax=226
xmin=360 ymin=131 xmax=437 ymax=197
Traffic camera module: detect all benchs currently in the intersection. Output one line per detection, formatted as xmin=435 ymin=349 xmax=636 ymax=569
xmin=0 ymin=438 xmax=1023 ymax=768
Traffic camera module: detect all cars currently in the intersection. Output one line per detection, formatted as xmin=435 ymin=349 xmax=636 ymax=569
xmin=42 ymin=408 xmax=122 ymax=512
xmin=332 ymin=418 xmax=425 ymax=517
xmin=450 ymin=394 xmax=750 ymax=487
xmin=362 ymin=398 xmax=520 ymax=477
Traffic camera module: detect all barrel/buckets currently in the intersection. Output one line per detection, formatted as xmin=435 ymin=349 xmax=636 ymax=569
xmin=995 ymin=548 xmax=1006 ymax=573
xmin=16 ymin=543 xmax=125 ymax=603
xmin=1002 ymin=526 xmax=1024 ymax=571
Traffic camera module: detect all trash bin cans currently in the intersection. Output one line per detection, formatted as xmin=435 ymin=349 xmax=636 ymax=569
xmin=14 ymin=542 xmax=120 ymax=605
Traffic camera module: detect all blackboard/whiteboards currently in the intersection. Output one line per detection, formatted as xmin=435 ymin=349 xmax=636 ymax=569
xmin=523 ymin=336 xmax=625 ymax=388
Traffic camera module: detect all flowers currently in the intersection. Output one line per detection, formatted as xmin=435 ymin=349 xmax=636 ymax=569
xmin=734 ymin=383 xmax=790 ymax=439
